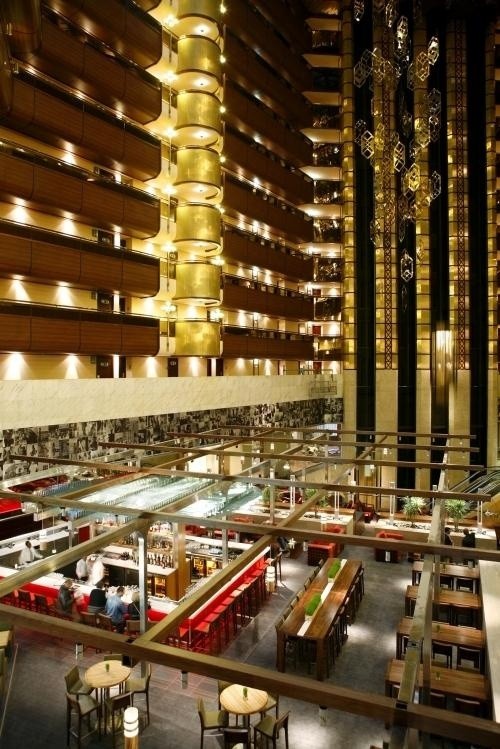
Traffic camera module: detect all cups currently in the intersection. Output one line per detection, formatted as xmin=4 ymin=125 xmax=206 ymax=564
xmin=105 ymin=663 xmax=109 ymax=674
xmin=442 ymin=561 xmax=445 ymax=571
xmin=242 ymin=686 xmax=249 ymax=700
xmin=436 ymin=624 xmax=439 ymax=633
xmin=435 ymin=669 xmax=441 ymax=680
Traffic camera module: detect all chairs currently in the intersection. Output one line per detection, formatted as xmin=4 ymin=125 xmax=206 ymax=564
xmin=223 ymin=728 xmax=250 ymax=749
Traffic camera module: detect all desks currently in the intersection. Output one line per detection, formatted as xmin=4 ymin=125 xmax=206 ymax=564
xmin=1 ymin=506 xmax=498 ymax=742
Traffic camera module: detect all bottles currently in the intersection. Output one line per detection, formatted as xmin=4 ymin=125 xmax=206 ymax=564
xmin=146 ymin=553 xmax=174 ymax=569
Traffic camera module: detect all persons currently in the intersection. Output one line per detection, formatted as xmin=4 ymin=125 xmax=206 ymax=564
xmin=1 ymin=399 xmax=343 ymax=477
xmin=462 ymin=528 xmax=476 ymax=566
xmin=444 ymin=527 xmax=453 ymax=545
xmin=87 ymin=580 xmax=106 ymax=614
xmin=278 ymin=536 xmax=289 ymax=550
xmin=56 ymin=579 xmax=80 ymax=614
xmin=19 ymin=541 xmax=44 ymax=565
xmin=75 ymin=555 xmax=89 ymax=582
xmin=105 ymin=586 xmax=128 ymax=634
